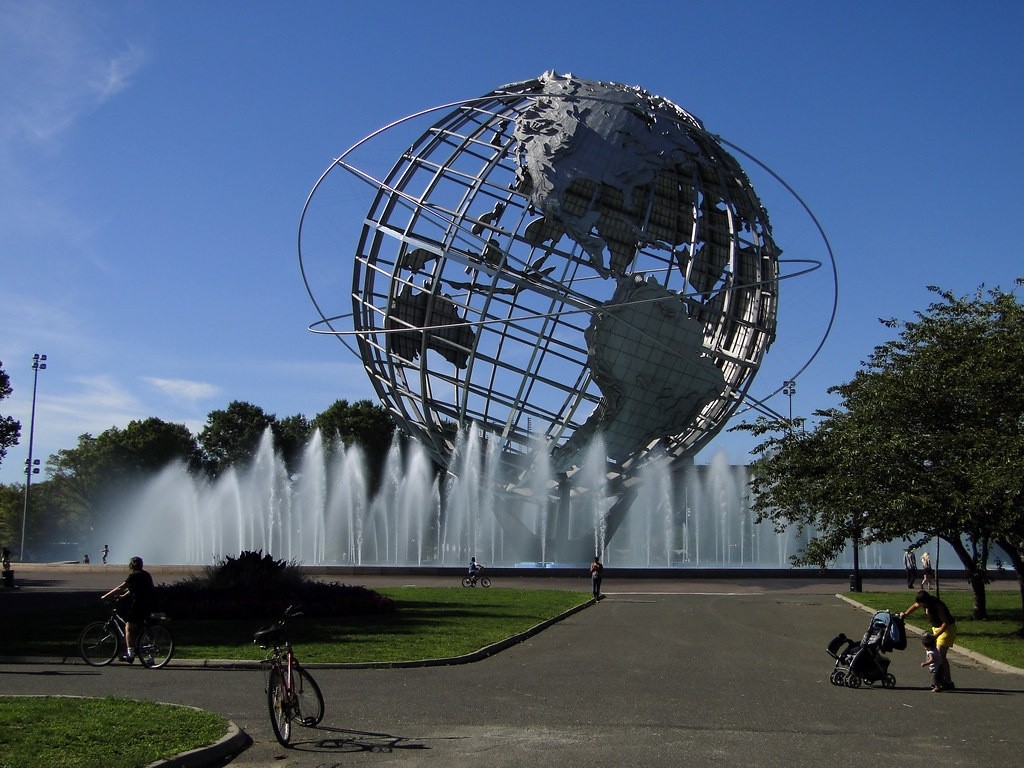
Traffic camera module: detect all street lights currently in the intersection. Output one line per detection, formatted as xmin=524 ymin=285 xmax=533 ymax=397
xmin=21 ymin=353 xmax=47 ymax=562
xmin=20 ymin=459 xmax=41 ymax=562
xmin=781 ymin=381 xmax=795 ymax=438
xmin=852 ymin=510 xmax=870 ymax=590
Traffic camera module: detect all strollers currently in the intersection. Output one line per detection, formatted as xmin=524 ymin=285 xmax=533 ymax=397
xmin=827 ymin=609 xmax=897 ymax=689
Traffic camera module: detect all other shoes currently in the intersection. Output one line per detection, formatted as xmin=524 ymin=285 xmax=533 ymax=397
xmin=117 ymin=654 xmax=135 ymax=664
xmin=931 ymin=688 xmax=941 ymax=692
xmin=931 ymin=682 xmax=954 ymax=689
xmin=928 ymin=587 xmax=933 ymax=590
xmin=920 ymin=584 xmax=923 ymax=589
xmin=596 ymin=600 xmax=599 ymax=603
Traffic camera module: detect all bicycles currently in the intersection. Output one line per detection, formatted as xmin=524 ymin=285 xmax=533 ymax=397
xmin=79 ymin=595 xmax=175 ymax=669
xmin=462 ymin=564 xmax=491 ymax=588
xmin=253 ymin=605 xmax=324 ymax=747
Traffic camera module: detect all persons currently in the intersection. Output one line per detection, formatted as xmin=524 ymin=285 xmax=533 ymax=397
xmin=101 ymin=556 xmax=155 ymax=664
xmin=83 ymin=555 xmax=89 ymax=564
xmin=903 ymin=545 xmax=917 ymax=589
xmin=100 ymin=544 xmax=109 ymax=564
xmin=1 ymin=547 xmax=12 ymax=571
xmin=920 ymin=551 xmax=933 ymax=589
xmin=469 ymin=557 xmax=478 ymax=587
xmin=899 ymin=589 xmax=957 ymax=693
xmin=590 ymin=557 xmax=603 ymax=602
